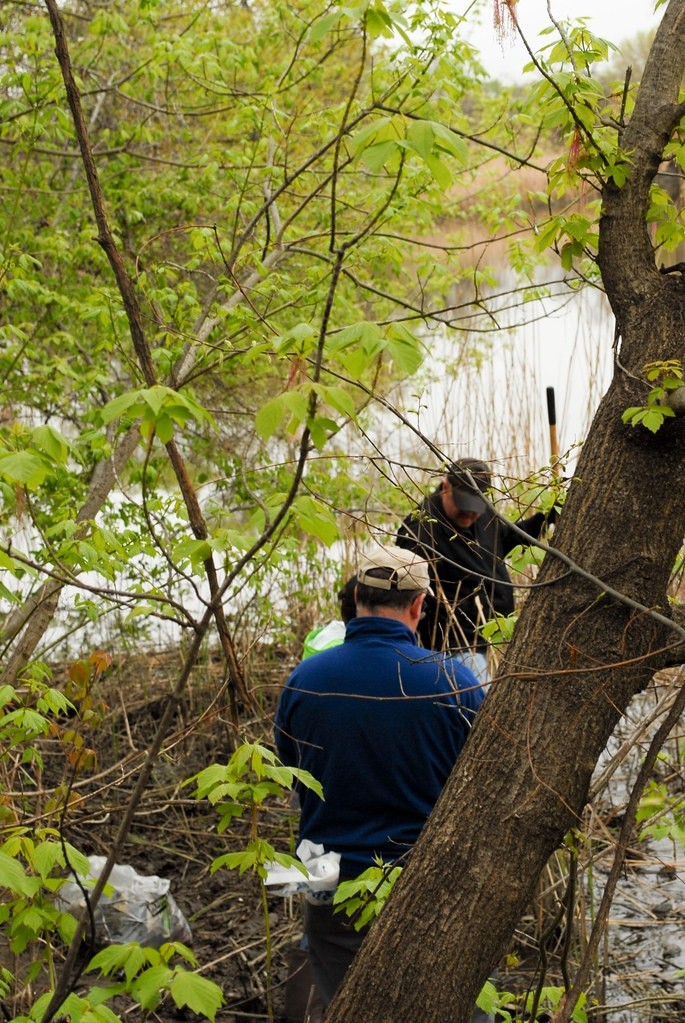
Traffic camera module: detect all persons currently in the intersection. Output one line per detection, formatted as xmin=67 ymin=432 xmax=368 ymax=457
xmin=301 ymin=575 xmax=358 ymax=658
xmin=397 ymin=459 xmax=548 ymax=697
xmin=275 ymin=546 xmax=499 ymax=1023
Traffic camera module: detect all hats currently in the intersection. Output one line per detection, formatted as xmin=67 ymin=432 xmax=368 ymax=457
xmin=448 ymin=458 xmax=491 ymax=513
xmin=357 ymin=546 xmax=435 ymax=596
